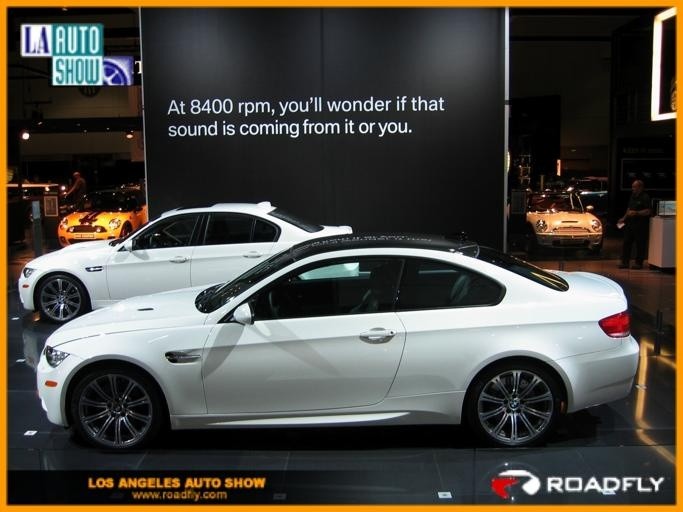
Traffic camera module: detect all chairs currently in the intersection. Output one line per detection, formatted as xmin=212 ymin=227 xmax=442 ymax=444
xmin=350 ymin=266 xmax=385 ymax=315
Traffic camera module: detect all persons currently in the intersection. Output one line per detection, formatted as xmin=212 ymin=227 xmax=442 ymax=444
xmin=616 ymin=180 xmax=652 ymax=269
xmin=65 ymin=172 xmax=85 ymax=204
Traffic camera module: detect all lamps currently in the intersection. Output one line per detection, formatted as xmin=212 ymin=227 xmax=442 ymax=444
xmin=125 ymin=130 xmax=133 ymax=139
xmin=19 ymin=129 xmax=30 ymax=140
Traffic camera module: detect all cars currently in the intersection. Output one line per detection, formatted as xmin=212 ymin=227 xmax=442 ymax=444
xmin=16 ymin=200 xmax=361 ymax=327
xmin=525 ymin=171 xmax=613 ymax=260
xmin=55 ymin=186 xmax=147 ymax=248
xmin=6 ymin=182 xmax=84 ymax=248
xmin=32 ymin=229 xmax=643 ymax=455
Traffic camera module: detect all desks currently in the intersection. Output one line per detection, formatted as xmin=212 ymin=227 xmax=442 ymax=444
xmin=647 ymin=216 xmax=676 ymax=269
